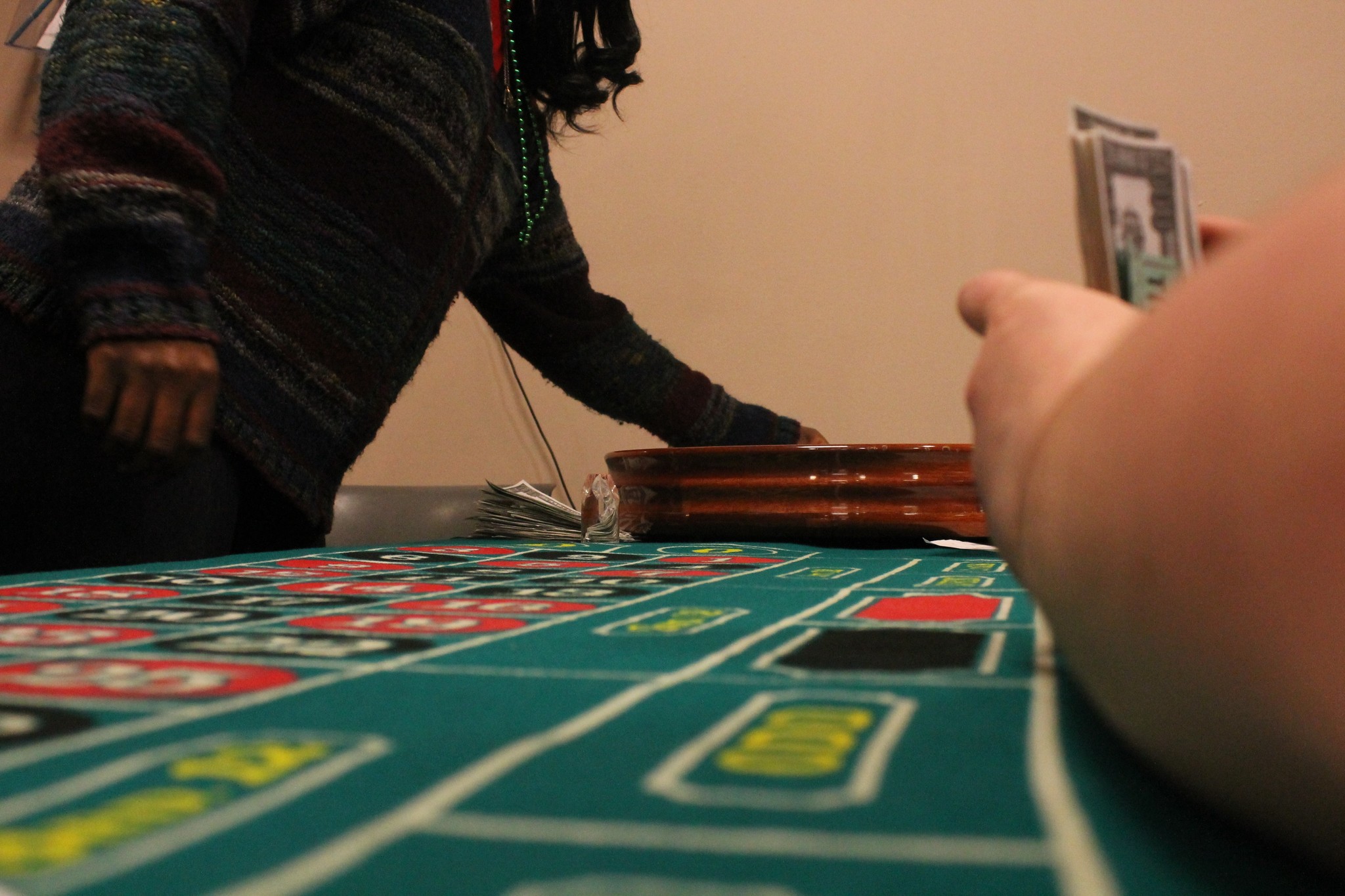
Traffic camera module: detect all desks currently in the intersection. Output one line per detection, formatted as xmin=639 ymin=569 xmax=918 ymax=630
xmin=0 ymin=537 xmax=1345 ymax=896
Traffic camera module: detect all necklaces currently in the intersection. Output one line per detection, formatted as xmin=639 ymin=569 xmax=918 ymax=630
xmin=507 ymin=0 xmax=550 ymax=247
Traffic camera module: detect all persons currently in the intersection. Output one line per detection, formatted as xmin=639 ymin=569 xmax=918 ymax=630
xmin=958 ymin=179 xmax=1345 ymax=836
xmin=0 ymin=0 xmax=830 ymax=576
